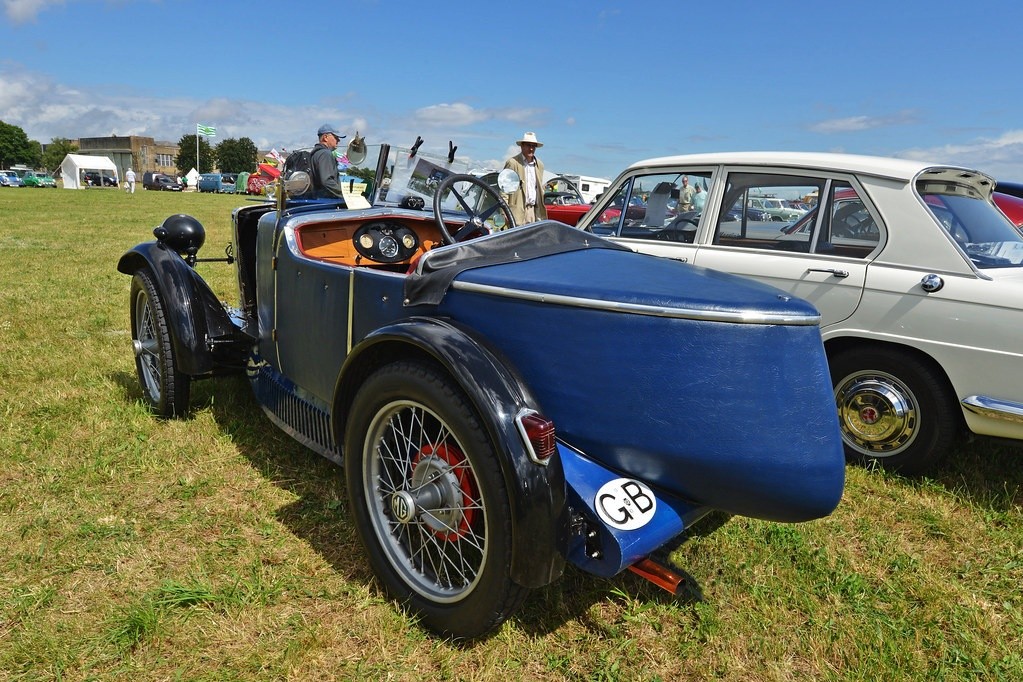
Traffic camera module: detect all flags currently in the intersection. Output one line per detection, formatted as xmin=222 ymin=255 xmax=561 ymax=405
xmin=197 ymin=124 xmax=216 ymax=137
xmin=267 ymin=147 xmax=286 ymax=165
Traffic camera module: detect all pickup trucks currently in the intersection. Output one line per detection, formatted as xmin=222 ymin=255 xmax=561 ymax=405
xmin=141 ymin=170 xmax=183 ymax=192
xmin=84 ymin=171 xmax=121 ymax=187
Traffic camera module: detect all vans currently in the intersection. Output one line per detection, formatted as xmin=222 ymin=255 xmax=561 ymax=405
xmin=195 ymin=163 xmax=281 ymax=196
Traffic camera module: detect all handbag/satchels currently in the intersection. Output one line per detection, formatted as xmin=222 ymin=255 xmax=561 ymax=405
xmin=124 ymin=182 xmax=130 ymax=189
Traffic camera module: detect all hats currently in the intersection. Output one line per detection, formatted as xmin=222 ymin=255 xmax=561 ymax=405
xmin=318 ymin=124 xmax=346 ymax=138
xmin=516 ymin=132 xmax=543 ymax=147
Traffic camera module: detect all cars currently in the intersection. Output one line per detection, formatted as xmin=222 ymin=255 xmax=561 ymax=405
xmin=572 ymin=148 xmax=1022 ymax=479
xmin=0 ymin=166 xmax=58 ymax=189
xmin=115 ymin=129 xmax=851 ymax=650
xmin=544 ymin=179 xmax=1023 ymax=257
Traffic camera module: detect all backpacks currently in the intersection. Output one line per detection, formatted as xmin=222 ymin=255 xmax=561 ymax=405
xmin=282 ymin=147 xmax=326 ymax=200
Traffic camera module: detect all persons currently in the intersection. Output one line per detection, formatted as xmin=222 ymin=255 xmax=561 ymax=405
xmin=690 ymin=181 xmax=708 ymax=216
xmin=499 ymin=132 xmax=548 ymax=231
xmin=124 ymin=168 xmax=136 ymax=194
xmin=679 ymin=176 xmax=696 ymax=213
xmin=281 ymin=123 xmax=348 ymax=210
xmin=176 ymin=173 xmax=188 ymax=191
